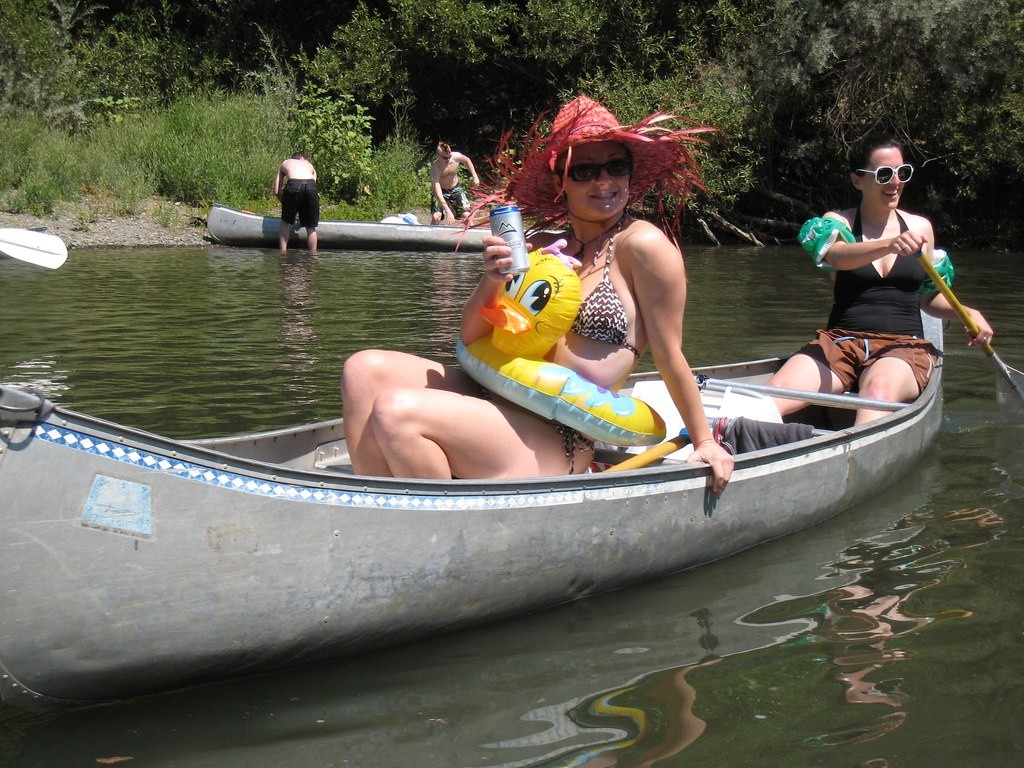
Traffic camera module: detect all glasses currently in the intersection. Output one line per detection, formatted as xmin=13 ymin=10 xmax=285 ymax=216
xmin=439 ymin=155 xmax=452 ymax=159
xmin=556 ymin=158 xmax=634 ymax=182
xmin=855 ymin=164 xmax=914 ymax=185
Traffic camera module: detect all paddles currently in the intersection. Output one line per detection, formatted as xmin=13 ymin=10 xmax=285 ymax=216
xmin=909 ymin=243 xmax=1024 ymax=428
xmin=0 ymin=227 xmax=70 ymax=273
xmin=598 ymin=425 xmax=693 ymax=476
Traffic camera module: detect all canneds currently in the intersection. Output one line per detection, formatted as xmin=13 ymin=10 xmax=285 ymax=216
xmin=489 ymin=205 xmax=530 ymax=276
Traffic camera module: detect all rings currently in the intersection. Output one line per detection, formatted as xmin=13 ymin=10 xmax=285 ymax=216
xmin=902 ymin=245 xmax=908 ymax=250
xmin=983 ymin=337 xmax=986 ymax=340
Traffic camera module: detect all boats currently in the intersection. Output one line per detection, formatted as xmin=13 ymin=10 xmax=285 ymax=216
xmin=199 ymin=200 xmax=565 ymax=254
xmin=0 ymin=305 xmax=944 ymax=714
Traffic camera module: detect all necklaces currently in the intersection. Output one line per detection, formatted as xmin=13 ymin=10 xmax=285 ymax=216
xmin=569 ymin=216 xmax=622 ymax=263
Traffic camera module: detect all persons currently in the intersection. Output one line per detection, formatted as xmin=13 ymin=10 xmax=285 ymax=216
xmin=430 ymin=142 xmax=480 ymax=226
xmin=274 ymin=151 xmax=320 ymax=253
xmin=341 ymin=97 xmax=734 ymax=499
xmin=765 ymin=134 xmax=993 ymax=428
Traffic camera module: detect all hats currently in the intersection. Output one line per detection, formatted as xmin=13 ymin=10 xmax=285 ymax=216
xmin=454 ymin=96 xmax=725 ymax=254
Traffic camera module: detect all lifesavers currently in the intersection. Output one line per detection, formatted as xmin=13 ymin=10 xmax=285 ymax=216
xmin=448 ymin=247 xmax=674 ymax=453
